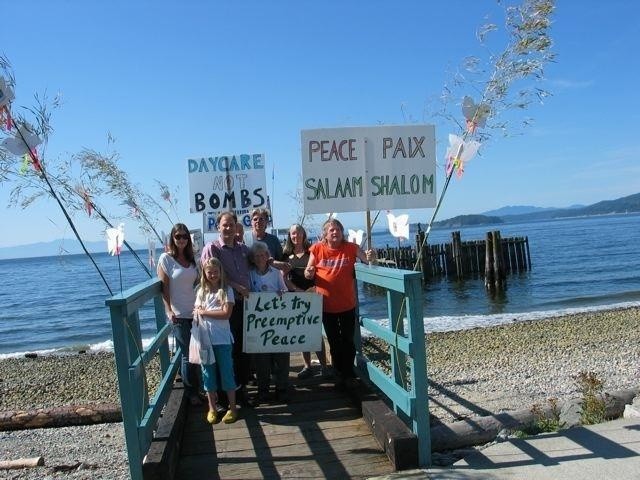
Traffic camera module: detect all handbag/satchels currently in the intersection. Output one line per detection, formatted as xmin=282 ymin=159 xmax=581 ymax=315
xmin=188 ymin=307 xmax=216 ymax=366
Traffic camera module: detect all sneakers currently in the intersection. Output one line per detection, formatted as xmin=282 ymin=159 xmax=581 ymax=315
xmin=298 ymin=367 xmax=315 ymax=378
xmin=320 ymin=367 xmax=330 ymax=380
xmin=258 ymin=388 xmax=292 ymax=402
xmin=247 ymin=378 xmax=258 ymax=386
xmin=190 ymin=389 xmax=209 ymax=407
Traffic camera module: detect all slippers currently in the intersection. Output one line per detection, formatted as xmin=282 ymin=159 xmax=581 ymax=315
xmin=206 ymin=403 xmax=241 ymax=425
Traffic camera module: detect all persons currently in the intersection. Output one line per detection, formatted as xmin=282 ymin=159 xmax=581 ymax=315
xmin=245 ymin=241 xmax=292 ymax=402
xmin=190 ymin=256 xmax=242 ymax=424
xmin=283 ymin=223 xmax=330 ymax=380
xmin=231 ymin=222 xmax=247 ymax=245
xmin=156 ymin=223 xmax=206 ymax=407
xmin=243 ymin=208 xmax=285 ymax=262
xmin=303 ymin=217 xmax=378 ymax=389
xmin=197 ymin=210 xmax=293 ymax=405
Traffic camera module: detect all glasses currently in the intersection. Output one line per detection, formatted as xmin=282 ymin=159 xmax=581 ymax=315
xmin=173 ymin=233 xmax=190 ymax=240
